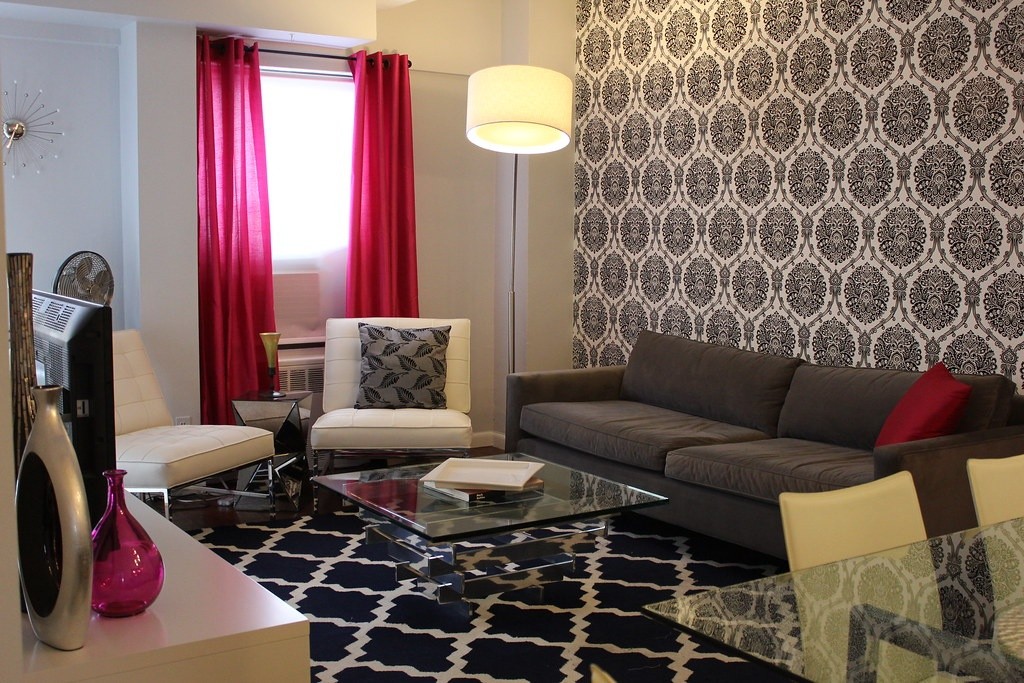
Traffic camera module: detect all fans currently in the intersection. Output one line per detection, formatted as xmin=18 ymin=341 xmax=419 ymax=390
xmin=53 ymin=251 xmax=114 ymax=306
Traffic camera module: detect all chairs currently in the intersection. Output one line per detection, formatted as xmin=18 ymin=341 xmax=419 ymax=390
xmin=778 ymin=471 xmax=969 ymax=682
xmin=113 ymin=329 xmax=277 ymax=532
xmin=311 ymin=318 xmax=473 ymax=514
xmin=967 ymin=456 xmax=1024 ymax=683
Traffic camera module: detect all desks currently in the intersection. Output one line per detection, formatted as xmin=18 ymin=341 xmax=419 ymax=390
xmin=645 ymin=516 xmax=1024 ymax=683
xmin=0 ymin=486 xmax=310 ymax=683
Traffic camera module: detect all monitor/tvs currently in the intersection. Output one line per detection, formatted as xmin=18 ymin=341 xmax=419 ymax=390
xmin=27 ymin=288 xmax=116 ymax=527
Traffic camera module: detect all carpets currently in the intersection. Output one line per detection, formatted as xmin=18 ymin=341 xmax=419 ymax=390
xmin=184 ymin=479 xmax=805 ymax=682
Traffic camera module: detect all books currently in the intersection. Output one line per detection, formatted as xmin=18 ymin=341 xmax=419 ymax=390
xmin=419 ymin=476 xmax=546 ymax=503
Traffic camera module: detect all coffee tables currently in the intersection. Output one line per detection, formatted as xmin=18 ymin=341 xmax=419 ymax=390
xmin=311 ymin=452 xmax=668 ymax=604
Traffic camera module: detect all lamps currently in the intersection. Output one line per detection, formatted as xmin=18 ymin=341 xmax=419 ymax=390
xmin=260 ymin=333 xmax=286 ymax=397
xmin=466 ymin=65 xmax=574 ymax=376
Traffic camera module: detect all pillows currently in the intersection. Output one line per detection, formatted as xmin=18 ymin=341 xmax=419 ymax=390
xmin=874 ymin=362 xmax=973 ymax=449
xmin=352 ymin=321 xmax=452 ymax=410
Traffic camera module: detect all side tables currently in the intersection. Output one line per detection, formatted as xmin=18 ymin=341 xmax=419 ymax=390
xmin=232 ymin=390 xmax=313 ymax=509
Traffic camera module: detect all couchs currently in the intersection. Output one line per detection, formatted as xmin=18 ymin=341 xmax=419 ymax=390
xmin=504 ymin=328 xmax=1024 ymax=582
